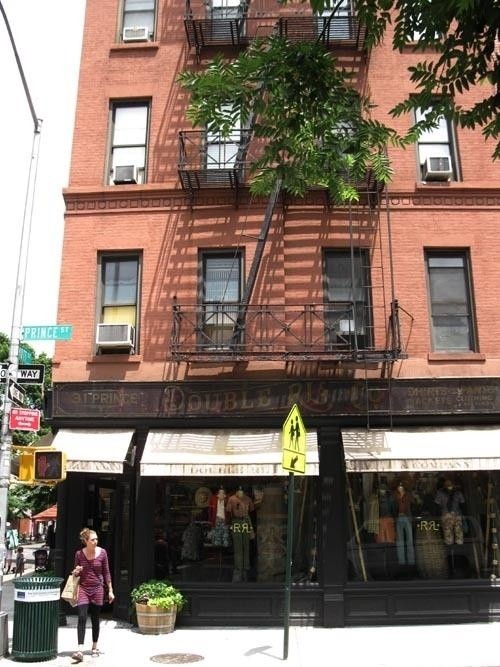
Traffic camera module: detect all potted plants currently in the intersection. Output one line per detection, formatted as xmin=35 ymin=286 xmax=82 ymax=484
xmin=130 ymin=577 xmax=188 ymax=634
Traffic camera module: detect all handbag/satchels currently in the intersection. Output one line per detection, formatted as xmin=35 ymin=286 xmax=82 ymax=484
xmin=61 ymin=575 xmax=81 ymax=607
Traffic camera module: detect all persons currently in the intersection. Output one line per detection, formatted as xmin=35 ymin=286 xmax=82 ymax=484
xmin=13 ymin=547 xmax=26 ymax=580
xmin=37 ymin=456 xmax=50 ymax=479
xmin=225 ymin=489 xmax=255 ymax=583
xmin=71 ymin=527 xmax=115 ymax=662
xmin=3 ymin=522 xmax=14 ymax=575
xmin=49 ymin=456 xmax=61 ymax=479
xmin=390 ymin=486 xmax=415 ymax=569
xmin=21 ymin=530 xmax=28 ymax=541
xmin=45 ymin=519 xmax=56 ymax=564
xmin=206 ymin=490 xmax=232 ymax=548
xmin=433 ymin=480 xmax=466 ymax=545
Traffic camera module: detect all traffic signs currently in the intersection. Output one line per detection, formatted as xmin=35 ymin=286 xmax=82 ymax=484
xmin=0 ymin=362 xmax=44 ymax=385
xmin=16 ymin=347 xmax=32 ymax=363
xmin=6 ymin=380 xmax=27 ymax=408
xmin=10 ymin=409 xmax=41 ymax=431
xmin=20 ymin=325 xmax=72 ymax=340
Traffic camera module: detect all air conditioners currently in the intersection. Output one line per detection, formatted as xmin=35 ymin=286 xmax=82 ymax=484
xmin=422 ymin=155 xmax=452 ymax=181
xmin=122 ymin=27 xmax=149 ymax=43
xmin=113 ymin=164 xmax=138 ymax=184
xmin=95 ymin=322 xmax=136 ymax=350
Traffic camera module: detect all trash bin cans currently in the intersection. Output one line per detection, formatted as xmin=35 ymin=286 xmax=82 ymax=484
xmin=12 ymin=577 xmax=64 ymax=662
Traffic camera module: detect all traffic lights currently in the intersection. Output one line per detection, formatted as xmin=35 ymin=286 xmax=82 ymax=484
xmin=30 ymin=448 xmax=67 ymax=487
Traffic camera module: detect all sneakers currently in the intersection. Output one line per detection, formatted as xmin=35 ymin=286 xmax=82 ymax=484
xmin=92 ymin=648 xmax=99 ymax=656
xmin=72 ymin=651 xmax=83 ymax=660
